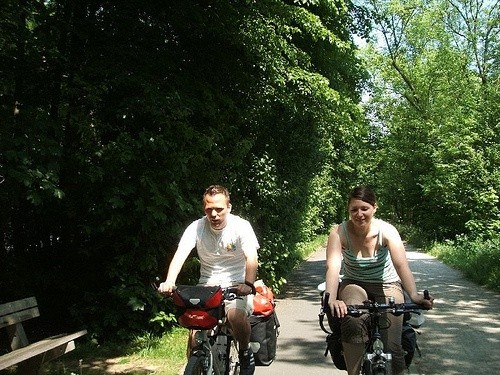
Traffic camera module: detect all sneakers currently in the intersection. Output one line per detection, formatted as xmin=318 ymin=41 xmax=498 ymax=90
xmin=239 ymin=344 xmax=255 ymax=375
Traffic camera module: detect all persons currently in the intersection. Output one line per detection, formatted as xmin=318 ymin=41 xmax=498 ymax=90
xmin=325 ymin=186 xmax=434 ymax=375
xmin=158 ymin=184 xmax=260 ymax=375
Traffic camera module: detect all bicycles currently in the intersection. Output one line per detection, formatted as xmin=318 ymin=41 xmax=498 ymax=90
xmin=315 ymin=273 xmax=433 ymax=375
xmin=149 ymin=275 xmax=281 ymax=375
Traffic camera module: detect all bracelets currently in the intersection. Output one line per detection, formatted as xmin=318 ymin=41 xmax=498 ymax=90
xmin=244 ymin=281 xmax=256 ymax=295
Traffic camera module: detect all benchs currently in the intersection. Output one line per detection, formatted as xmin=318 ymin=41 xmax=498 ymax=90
xmin=0 ymin=297 xmax=87 ymax=375
